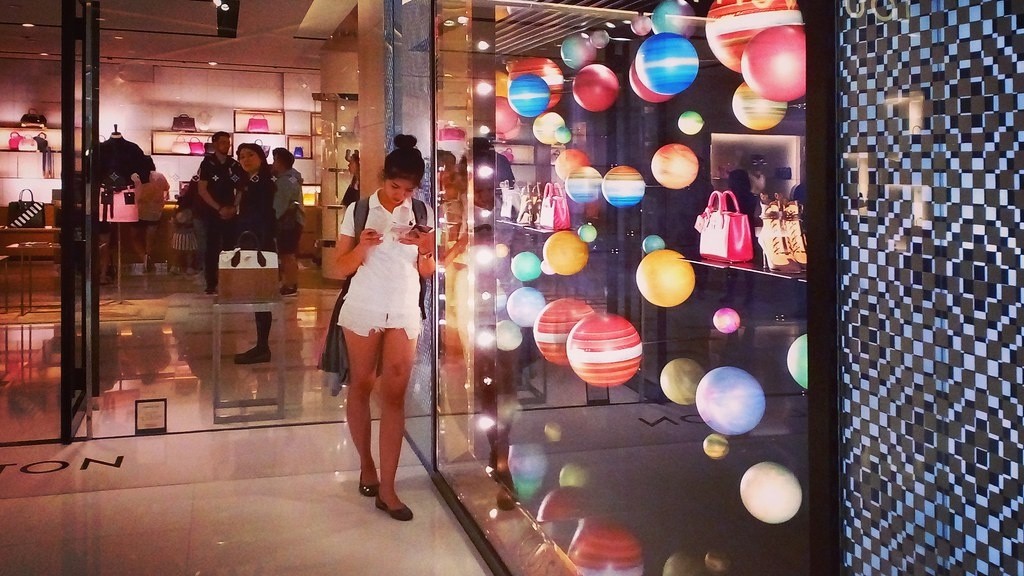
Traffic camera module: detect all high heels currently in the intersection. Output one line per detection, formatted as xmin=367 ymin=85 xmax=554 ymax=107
xmin=757 ymin=198 xmax=809 ymax=273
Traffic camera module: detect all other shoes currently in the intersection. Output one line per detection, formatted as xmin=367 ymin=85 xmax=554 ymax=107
xmin=203 ymin=286 xmax=218 ymax=296
xmin=374 ymin=494 xmax=413 ymax=520
xmin=359 ymin=469 xmax=378 ymax=496
xmin=233 ymin=343 xmax=271 ymax=365
xmin=280 ymin=285 xmax=297 ymax=298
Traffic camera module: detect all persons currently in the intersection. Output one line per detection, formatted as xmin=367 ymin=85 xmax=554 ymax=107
xmin=331 ymin=134 xmax=436 ymax=521
xmin=342 ymin=153 xmax=360 ymax=209
xmin=228 ymin=143 xmax=276 ymax=365
xmin=130 ymin=154 xmax=170 ymax=274
xmin=447 ymin=139 xmax=536 ymax=511
xmin=99 ymin=132 xmax=145 ymax=223
xmin=171 ymin=132 xmax=304 ymax=296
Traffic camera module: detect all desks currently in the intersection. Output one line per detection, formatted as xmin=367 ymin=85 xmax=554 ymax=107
xmin=0 ymin=225 xmax=62 ymax=256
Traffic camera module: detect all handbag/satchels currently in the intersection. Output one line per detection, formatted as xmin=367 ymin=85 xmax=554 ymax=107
xmin=293 ymin=144 xmax=303 ymax=157
xmin=18 ymin=134 xmax=38 ymax=151
xmin=216 ymin=230 xmax=281 ymax=303
xmin=8 ymin=131 xmax=24 ymax=150
xmin=187 ymin=137 xmax=205 ymax=154
xmin=7 ymin=189 xmax=45 ymax=228
xmin=252 ymin=139 xmax=270 ymax=159
xmin=247 ymin=113 xmax=270 ymax=133
xmin=171 ymin=136 xmax=191 ymax=155
xmin=33 ymin=132 xmax=48 ymax=151
xmin=171 ymin=114 xmax=196 ymax=133
xmin=498 ymin=177 xmax=573 ymax=230
xmin=20 ymin=108 xmax=47 ymax=128
xmin=204 ymin=136 xmax=216 ymax=155
xmin=697 ymin=190 xmax=755 ymax=265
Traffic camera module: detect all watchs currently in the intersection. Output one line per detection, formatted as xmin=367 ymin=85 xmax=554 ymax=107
xmin=418 ymin=249 xmax=433 ymax=260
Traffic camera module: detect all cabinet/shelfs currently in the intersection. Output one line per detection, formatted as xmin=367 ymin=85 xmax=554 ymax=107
xmin=323 ymin=93 xmax=360 ymax=281
xmin=212 ymin=294 xmax=285 ymax=424
xmin=0 ymin=127 xmax=63 ymax=154
xmin=150 ymin=108 xmax=321 ymax=160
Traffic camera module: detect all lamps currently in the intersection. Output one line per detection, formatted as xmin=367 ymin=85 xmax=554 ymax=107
xmin=99 ymin=185 xmax=140 ymax=306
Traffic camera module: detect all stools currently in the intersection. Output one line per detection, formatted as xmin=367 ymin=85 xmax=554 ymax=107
xmin=0 ymin=255 xmax=10 ymax=314
xmin=5 ymin=241 xmax=62 ymax=316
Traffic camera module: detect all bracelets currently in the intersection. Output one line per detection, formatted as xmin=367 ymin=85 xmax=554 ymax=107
xmin=218 ymin=205 xmax=223 ymax=212
